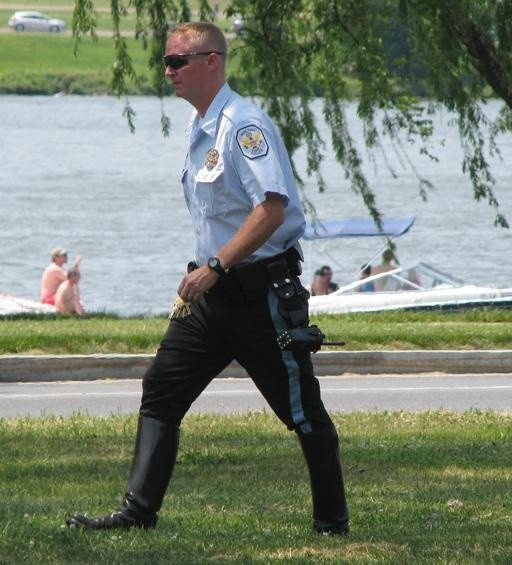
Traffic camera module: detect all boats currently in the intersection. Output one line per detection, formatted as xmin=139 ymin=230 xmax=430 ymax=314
xmin=303 ymin=216 xmax=511 ymax=314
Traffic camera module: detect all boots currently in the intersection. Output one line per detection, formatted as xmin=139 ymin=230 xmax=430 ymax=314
xmin=66 ymin=414 xmax=181 ymax=533
xmin=300 ymin=422 xmax=351 ymax=535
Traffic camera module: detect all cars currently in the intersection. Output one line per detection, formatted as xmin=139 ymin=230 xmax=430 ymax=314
xmin=7 ymin=10 xmax=66 ymax=32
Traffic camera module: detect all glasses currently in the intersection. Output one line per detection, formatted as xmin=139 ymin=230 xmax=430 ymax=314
xmin=163 ymin=51 xmax=224 ymax=70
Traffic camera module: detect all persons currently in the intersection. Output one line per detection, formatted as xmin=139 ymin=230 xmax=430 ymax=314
xmin=66 ymin=21 xmax=350 ymax=537
xmin=41 ymin=248 xmax=82 ymax=304
xmin=54 ymin=267 xmax=85 ymax=315
xmin=310 ymin=250 xmax=421 ymax=296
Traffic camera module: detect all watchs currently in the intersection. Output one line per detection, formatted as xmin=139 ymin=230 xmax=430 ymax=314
xmin=207 ymin=257 xmax=225 ymax=278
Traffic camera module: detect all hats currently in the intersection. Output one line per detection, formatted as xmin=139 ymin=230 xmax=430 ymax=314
xmin=52 ymin=248 xmax=66 ymax=256
